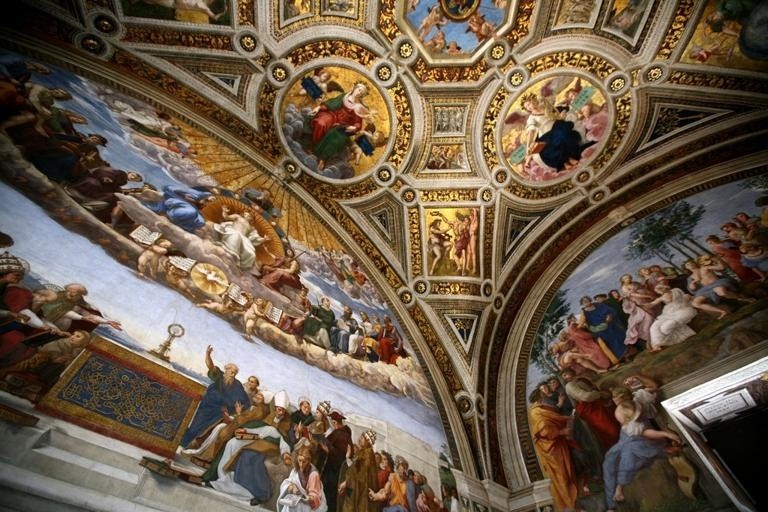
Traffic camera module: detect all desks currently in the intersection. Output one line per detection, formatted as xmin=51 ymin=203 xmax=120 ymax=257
xmin=35 ymin=335 xmax=210 ymax=460
